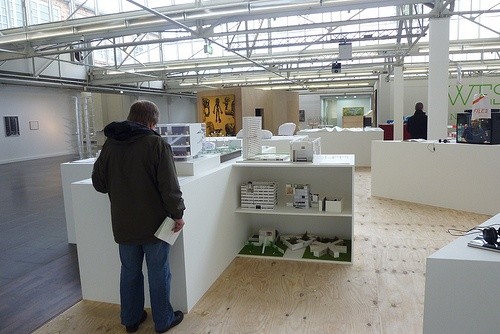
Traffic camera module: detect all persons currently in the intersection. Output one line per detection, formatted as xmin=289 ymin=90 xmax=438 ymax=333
xmin=88 ymin=98 xmax=187 ymax=334
xmin=406 ymin=102 xmax=429 ymax=141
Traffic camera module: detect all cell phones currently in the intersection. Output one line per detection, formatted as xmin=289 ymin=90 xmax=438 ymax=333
xmin=467 ymin=233 xmax=500 ymax=253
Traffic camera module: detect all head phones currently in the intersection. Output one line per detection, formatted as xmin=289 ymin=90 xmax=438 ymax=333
xmin=480 ymin=224 xmax=500 ymax=244
xmin=439 ymin=139 xmax=450 ymax=143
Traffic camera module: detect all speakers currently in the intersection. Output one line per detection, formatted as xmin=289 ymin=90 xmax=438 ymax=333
xmin=455 ymin=112 xmax=500 ymax=145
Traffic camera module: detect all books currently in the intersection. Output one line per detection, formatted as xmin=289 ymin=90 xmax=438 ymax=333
xmin=153 ymin=216 xmax=183 ymax=246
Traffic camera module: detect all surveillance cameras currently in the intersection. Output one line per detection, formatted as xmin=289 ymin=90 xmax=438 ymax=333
xmin=80 ymin=36 xmax=86 ymax=41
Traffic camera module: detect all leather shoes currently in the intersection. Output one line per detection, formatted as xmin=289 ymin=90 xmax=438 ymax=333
xmin=125 ymin=310 xmax=147 ymax=333
xmin=158 ymin=310 xmax=185 ymax=334
